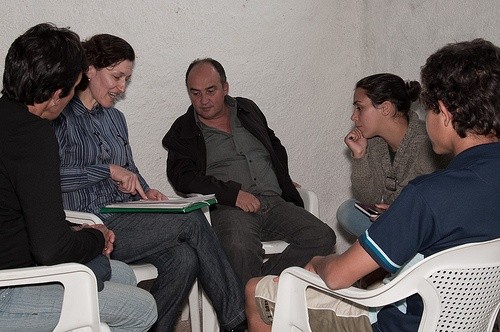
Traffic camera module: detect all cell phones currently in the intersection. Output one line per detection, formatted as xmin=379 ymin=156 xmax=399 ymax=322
xmin=367 ymin=207 xmax=383 ymax=215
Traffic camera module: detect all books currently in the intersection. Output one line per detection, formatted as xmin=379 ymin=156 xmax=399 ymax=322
xmin=107 ymin=192 xmax=215 ymax=209
xmin=354 ymin=202 xmax=380 ymax=218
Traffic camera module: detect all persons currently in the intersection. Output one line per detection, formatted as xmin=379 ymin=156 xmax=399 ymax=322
xmin=245 ymin=38 xmax=500 ymax=332
xmin=0 ymin=23 xmax=158 ymax=332
xmin=51 ymin=33 xmax=248 ymax=332
xmin=336 ymin=73 xmax=455 ymax=236
xmin=162 ymin=58 xmax=336 ymax=300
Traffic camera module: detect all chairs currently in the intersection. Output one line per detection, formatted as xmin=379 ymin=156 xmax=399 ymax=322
xmin=0 ymin=187 xmax=500 ymax=332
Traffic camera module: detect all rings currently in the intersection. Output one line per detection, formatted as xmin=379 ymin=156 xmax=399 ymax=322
xmin=104 ymin=247 xmax=107 ymax=251
